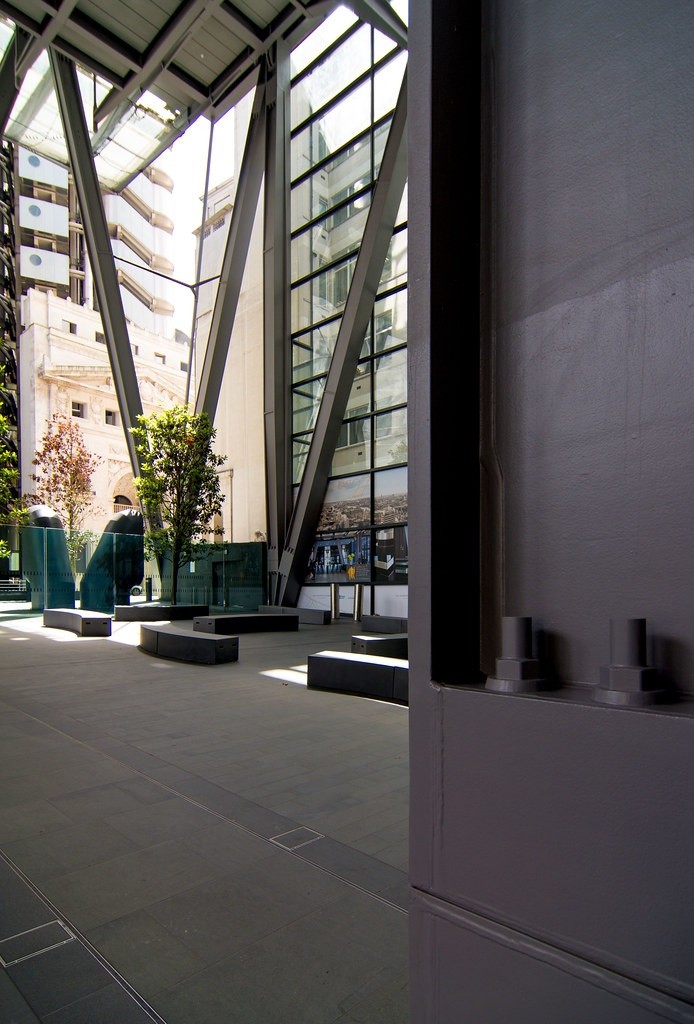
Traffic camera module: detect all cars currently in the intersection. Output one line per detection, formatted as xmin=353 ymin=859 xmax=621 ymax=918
xmin=129 ymin=585 xmax=143 ymax=596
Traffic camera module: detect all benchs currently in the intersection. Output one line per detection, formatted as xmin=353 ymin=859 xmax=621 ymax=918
xmin=43 ymin=608 xmax=111 ymax=636
xmin=192 ymin=614 xmax=299 ymax=635
xmin=308 ymin=650 xmax=410 ymax=706
xmin=114 ymin=604 xmax=209 ymax=621
xmin=258 ymin=604 xmax=332 ymax=625
xmin=136 ymin=624 xmax=240 ymax=665
xmin=350 ymin=613 xmax=409 ymax=660
